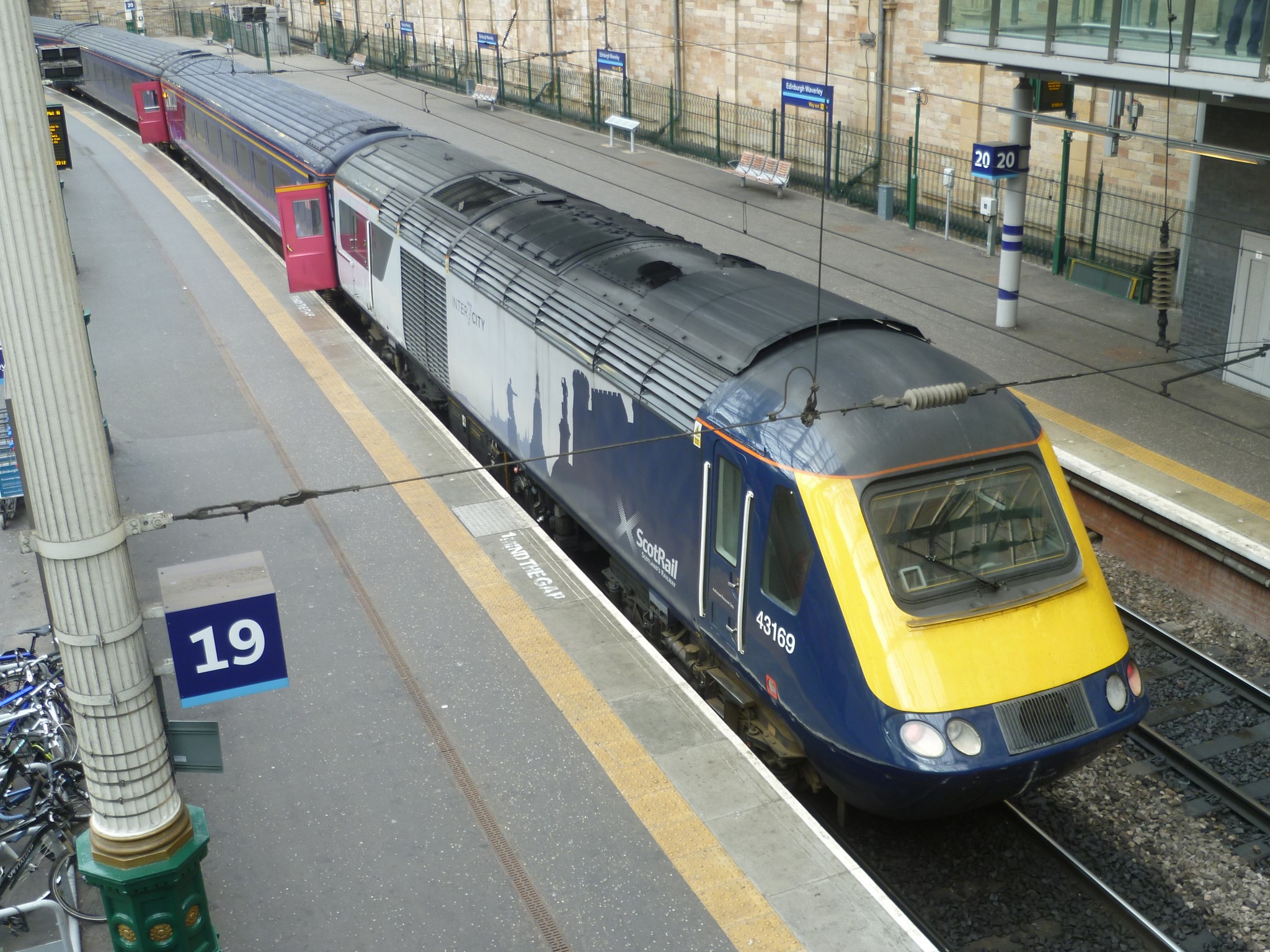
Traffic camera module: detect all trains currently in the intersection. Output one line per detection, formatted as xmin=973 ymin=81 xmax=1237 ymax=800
xmin=29 ymin=13 xmax=1145 ymax=822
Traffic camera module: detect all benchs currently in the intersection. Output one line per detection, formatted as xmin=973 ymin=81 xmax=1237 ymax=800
xmin=467 ymin=84 xmax=498 ymax=111
xmin=729 ymin=150 xmax=793 ymax=199
xmin=206 ymin=31 xmax=214 ymax=45
xmin=224 ymin=38 xmax=235 ymax=54
xmin=350 ymin=53 xmax=367 ymax=74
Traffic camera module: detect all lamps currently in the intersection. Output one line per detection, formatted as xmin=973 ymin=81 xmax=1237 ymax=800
xmin=1163 ymin=142 xmax=1266 ymax=165
xmin=1033 ymin=119 xmax=1120 ymax=138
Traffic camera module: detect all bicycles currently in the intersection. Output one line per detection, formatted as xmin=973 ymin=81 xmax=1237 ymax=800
xmin=0 ymin=620 xmax=119 ymax=932
